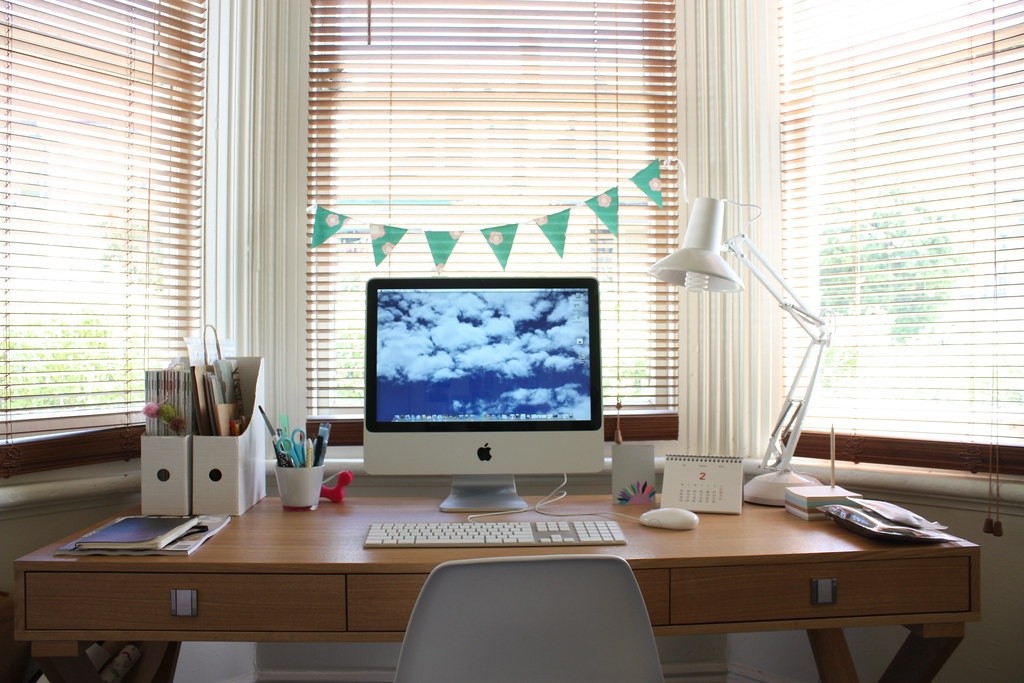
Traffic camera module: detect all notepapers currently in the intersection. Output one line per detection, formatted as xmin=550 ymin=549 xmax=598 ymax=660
xmin=784 ymin=485 xmax=863 ymax=521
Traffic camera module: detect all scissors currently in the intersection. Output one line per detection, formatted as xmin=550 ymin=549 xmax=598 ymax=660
xmin=276 ymin=428 xmax=306 ymax=468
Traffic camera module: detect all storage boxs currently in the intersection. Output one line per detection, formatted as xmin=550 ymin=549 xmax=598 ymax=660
xmin=191 ymin=355 xmax=267 ymax=517
xmin=140 ymin=358 xmax=192 ymax=516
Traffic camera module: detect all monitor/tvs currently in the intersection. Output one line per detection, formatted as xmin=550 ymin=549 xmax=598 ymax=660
xmin=362 ymin=279 xmax=606 ymax=511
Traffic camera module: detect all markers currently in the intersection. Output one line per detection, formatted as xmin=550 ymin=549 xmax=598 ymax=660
xmin=258 ymin=405 xmax=331 ymax=467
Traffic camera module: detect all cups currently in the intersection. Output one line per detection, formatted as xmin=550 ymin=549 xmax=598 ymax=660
xmin=274 ymin=465 xmax=325 ymax=511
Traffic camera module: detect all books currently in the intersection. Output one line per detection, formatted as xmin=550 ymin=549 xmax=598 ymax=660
xmin=145 ymin=359 xmax=241 ymax=436
xmin=54 ymin=513 xmax=232 ymax=556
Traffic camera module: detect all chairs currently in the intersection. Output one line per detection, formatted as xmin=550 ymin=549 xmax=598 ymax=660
xmin=392 ymin=555 xmax=664 ymax=683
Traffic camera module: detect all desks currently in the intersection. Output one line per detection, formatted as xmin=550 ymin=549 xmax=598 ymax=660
xmin=13 ymin=496 xmax=982 ymax=682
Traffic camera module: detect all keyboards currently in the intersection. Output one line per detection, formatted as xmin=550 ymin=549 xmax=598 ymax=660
xmin=363 ymin=520 xmax=627 ymax=546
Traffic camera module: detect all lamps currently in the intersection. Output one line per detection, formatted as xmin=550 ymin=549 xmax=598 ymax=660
xmin=645 ymin=198 xmax=832 ymax=506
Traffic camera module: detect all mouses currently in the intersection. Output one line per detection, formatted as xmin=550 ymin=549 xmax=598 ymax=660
xmin=639 ymin=507 xmax=699 ymax=529
